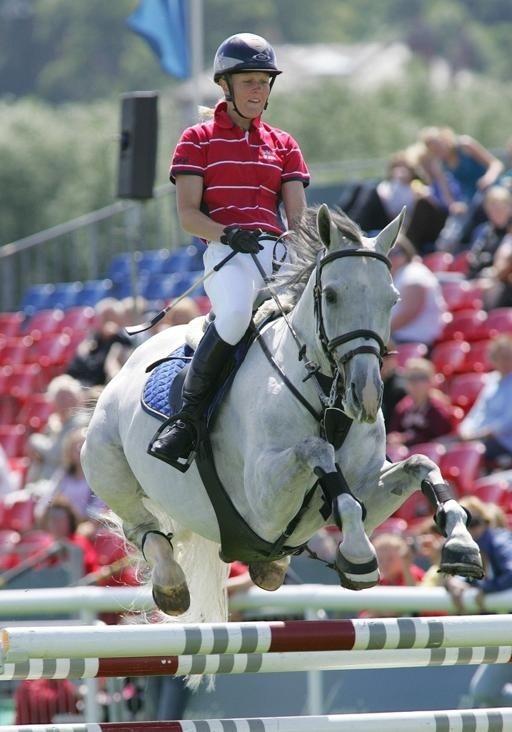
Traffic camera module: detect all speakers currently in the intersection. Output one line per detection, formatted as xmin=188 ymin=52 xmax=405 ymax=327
xmin=117 ymin=97 xmax=157 ymax=199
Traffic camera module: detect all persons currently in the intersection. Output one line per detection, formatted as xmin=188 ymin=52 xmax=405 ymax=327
xmin=1 ymin=296 xmax=248 ymax=723
xmin=357 ymin=125 xmax=512 ymax=623
xmin=151 ymin=32 xmax=311 ymax=465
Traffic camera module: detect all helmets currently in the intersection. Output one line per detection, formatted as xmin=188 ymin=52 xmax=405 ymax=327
xmin=212 ymin=33 xmax=283 ymax=82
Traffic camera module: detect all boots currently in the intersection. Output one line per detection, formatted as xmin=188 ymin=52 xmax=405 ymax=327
xmin=151 ymin=323 xmax=238 ymax=463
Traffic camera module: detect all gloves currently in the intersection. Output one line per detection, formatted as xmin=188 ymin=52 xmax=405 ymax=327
xmin=219 ymin=223 xmax=264 ymax=255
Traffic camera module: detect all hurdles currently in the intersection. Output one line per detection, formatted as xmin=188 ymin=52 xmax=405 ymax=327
xmin=0 ymin=581 xmax=512 ymax=732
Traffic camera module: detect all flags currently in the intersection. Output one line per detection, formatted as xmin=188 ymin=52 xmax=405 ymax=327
xmin=124 ymin=0 xmax=196 ymax=82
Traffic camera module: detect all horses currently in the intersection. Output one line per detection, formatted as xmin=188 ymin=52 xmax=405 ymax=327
xmin=64 ymin=201 xmax=487 ymax=696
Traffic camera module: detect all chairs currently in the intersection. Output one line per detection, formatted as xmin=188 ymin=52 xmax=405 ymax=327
xmin=2 ymin=236 xmax=511 ymax=568
